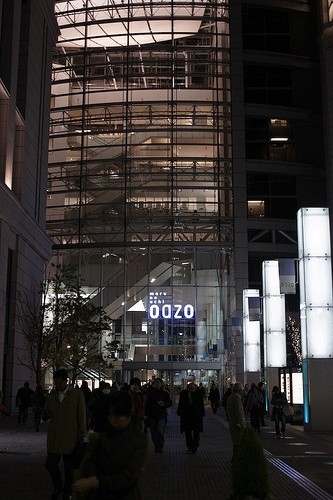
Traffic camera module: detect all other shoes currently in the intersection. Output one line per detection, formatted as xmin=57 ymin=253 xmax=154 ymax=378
xmin=50 ymin=483 xmax=63 ymax=500
xmin=63 ymin=490 xmax=70 ymax=500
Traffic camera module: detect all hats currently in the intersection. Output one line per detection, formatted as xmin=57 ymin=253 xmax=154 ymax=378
xmin=103 ymin=390 xmax=134 ymax=417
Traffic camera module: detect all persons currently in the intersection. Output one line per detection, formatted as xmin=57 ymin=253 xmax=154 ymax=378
xmin=226 ymin=383 xmax=247 ymax=462
xmin=144 ymin=379 xmax=172 ymax=453
xmin=270 ymin=386 xmax=288 ymax=439
xmin=80 ymin=382 xmax=234 ymax=404
xmin=248 ymin=384 xmax=263 ymax=428
xmin=32 ymin=386 xmax=45 ymax=431
xmin=177 ymin=382 xmax=206 ymax=452
xmin=241 ymin=384 xmax=249 ymax=411
xmin=15 ymin=382 xmax=33 ymax=424
xmin=209 ymin=384 xmax=219 ymax=414
xmin=223 ymin=388 xmax=233 ymax=420
xmin=43 ymin=368 xmax=85 ymax=500
xmin=258 ymin=382 xmax=269 ymax=427
xmin=73 ymin=397 xmax=140 ymax=500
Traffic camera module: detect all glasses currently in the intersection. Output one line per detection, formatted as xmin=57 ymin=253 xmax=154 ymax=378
xmin=53 ymin=381 xmax=65 ymax=385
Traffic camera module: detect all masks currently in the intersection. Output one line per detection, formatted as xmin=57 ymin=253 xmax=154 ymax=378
xmin=103 ymin=389 xmax=111 ymax=394
